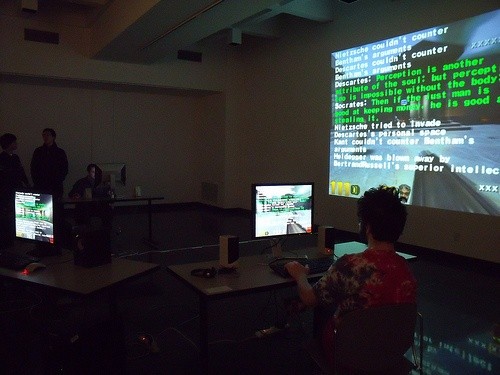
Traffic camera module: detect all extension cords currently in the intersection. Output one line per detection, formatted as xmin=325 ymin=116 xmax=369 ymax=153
xmin=256 ymin=327 xmax=278 ymax=338
xmin=137 ymin=335 xmax=159 ymax=353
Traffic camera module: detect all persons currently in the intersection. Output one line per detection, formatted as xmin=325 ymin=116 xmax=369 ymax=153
xmin=69 ymin=164 xmax=108 ymax=200
xmin=284 ymin=185 xmax=418 ymax=375
xmin=0 ymin=133 xmax=28 ymax=241
xmin=30 ymin=129 xmax=68 ymax=200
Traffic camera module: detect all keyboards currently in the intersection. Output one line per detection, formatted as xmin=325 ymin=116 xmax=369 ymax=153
xmin=0 ymin=245 xmax=40 ymax=271
xmin=270 ymin=252 xmax=336 ymax=278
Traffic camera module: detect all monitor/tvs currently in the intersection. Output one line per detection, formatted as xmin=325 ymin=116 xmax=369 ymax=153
xmin=10 ymin=188 xmax=56 ymax=254
xmin=251 ymin=182 xmax=315 ymax=259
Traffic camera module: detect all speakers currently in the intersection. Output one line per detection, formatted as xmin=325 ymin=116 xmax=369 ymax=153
xmin=317 ymin=225 xmax=336 ymax=256
xmin=218 ymin=234 xmax=241 ymax=274
xmin=73 ymin=222 xmax=113 ymax=267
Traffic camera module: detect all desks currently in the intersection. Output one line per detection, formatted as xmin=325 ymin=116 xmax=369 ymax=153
xmin=0 ymin=251 xmax=162 ymax=375
xmin=167 ymin=241 xmax=418 ymax=375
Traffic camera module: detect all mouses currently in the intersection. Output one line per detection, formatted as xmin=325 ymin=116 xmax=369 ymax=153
xmin=24 ymin=262 xmax=48 ymax=273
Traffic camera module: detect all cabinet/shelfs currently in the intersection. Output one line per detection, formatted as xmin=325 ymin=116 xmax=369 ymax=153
xmin=56 ymin=195 xmax=164 ymax=250
